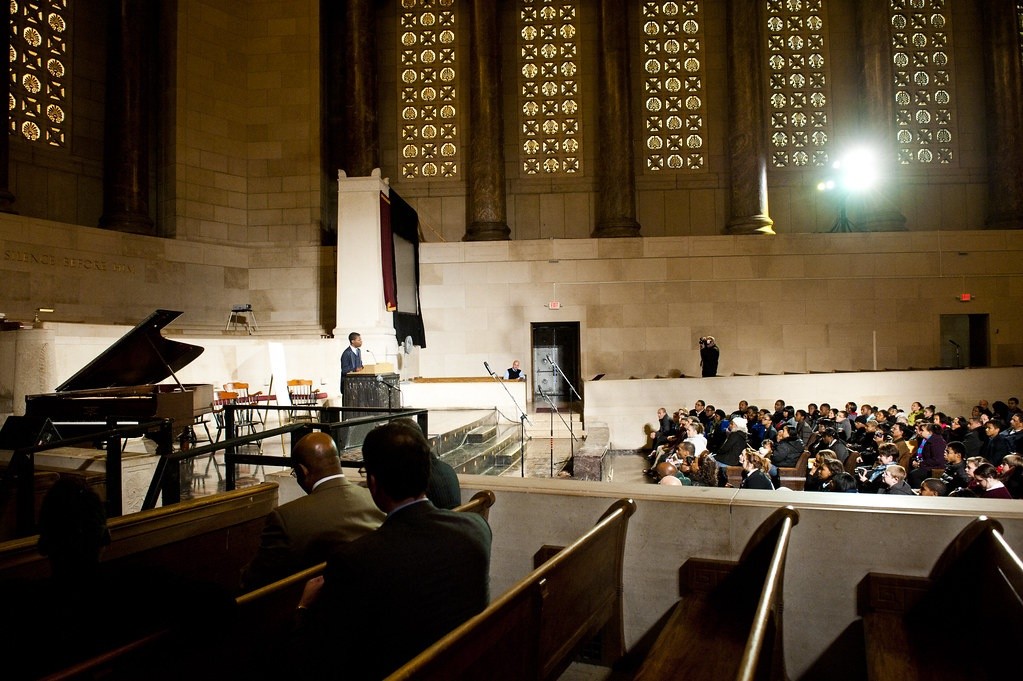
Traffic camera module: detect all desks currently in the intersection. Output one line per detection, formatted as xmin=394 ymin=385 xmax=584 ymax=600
xmin=226 ymin=310 xmax=259 ymax=336
xmin=343 ymin=374 xmax=400 ymax=420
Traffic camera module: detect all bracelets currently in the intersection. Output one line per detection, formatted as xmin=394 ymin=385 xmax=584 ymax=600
xmin=298 ymin=604 xmax=304 ymax=608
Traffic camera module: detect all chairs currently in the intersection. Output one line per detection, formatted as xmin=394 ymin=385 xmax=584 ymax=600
xmin=213 ymin=380 xmax=318 ymax=446
xmin=726 ymin=450 xmax=808 ymax=489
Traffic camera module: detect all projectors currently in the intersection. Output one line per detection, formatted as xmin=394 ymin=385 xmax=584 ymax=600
xmin=233 ymin=303 xmax=252 ymax=310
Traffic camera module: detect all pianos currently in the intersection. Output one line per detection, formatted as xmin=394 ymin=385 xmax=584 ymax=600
xmin=1 ymin=307 xmax=217 ymax=518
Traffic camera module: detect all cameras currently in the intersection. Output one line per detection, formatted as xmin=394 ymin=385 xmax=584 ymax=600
xmin=699 ymin=338 xmax=711 ymax=345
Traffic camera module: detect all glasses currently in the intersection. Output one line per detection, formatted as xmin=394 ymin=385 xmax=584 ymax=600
xmin=290 ymin=466 xmax=300 ymax=478
xmin=946 ymin=449 xmax=950 ymax=454
xmin=358 ymin=464 xmax=373 ymax=478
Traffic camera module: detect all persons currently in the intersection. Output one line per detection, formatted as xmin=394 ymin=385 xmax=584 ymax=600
xmin=650 ymin=397 xmax=1023 ymax=499
xmin=240 ymin=432 xmax=387 ymax=593
xmin=699 ymin=336 xmax=720 ymax=378
xmin=283 ymin=422 xmax=493 ymax=681
xmin=0 ymin=478 xmax=156 ymax=681
xmin=340 ymin=333 xmax=364 ymax=395
xmin=393 ymin=418 xmax=462 ymax=510
xmin=504 ymin=360 xmax=524 ymax=379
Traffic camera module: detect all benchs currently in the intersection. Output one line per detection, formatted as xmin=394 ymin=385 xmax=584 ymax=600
xmin=0 ymin=483 xmax=1023 ymax=681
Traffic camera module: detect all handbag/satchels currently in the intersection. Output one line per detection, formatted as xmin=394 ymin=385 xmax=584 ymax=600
xmin=642 ymin=468 xmax=653 ymax=473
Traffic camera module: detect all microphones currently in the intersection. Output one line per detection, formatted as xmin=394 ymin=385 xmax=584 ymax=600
xmin=537 ymin=385 xmax=545 ymax=401
xmin=376 ymin=375 xmax=400 ymax=392
xmin=949 ymin=339 xmax=960 ymax=348
xmin=367 ymin=349 xmax=378 ymax=365
xmin=546 ymin=354 xmax=554 ymax=365
xmin=484 ymin=362 xmax=496 ymax=380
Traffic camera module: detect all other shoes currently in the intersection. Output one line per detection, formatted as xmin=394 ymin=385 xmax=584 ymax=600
xmin=648 ymin=450 xmax=656 ymax=458
